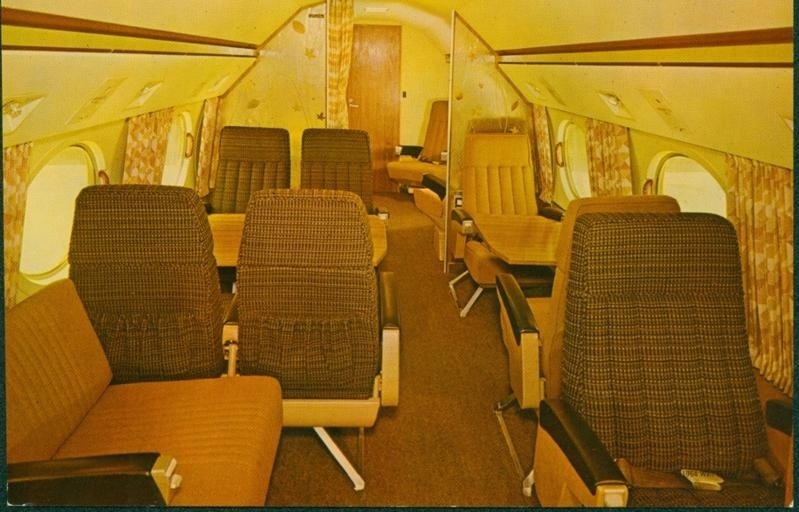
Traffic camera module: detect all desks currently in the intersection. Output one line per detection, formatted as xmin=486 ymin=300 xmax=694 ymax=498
xmin=449 ymin=214 xmax=562 ymax=318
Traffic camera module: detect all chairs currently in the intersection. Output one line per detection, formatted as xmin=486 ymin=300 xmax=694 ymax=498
xmin=532 ymin=212 xmax=786 ymax=508
xmin=406 ymin=173 xmax=448 ymax=261
xmin=448 ymin=132 xmax=565 ymax=265
xmin=386 ymin=100 xmax=448 ymax=194
xmin=492 ymin=193 xmax=683 ymax=498
